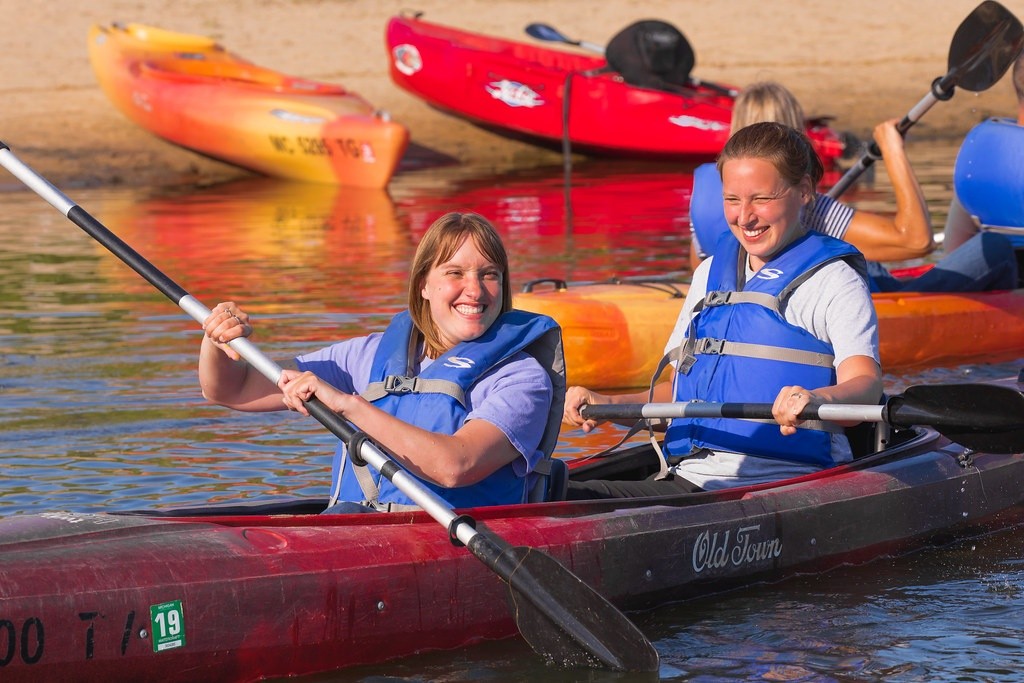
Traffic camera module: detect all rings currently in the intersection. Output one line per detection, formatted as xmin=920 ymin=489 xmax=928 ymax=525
xmin=233 ymin=315 xmax=242 ymax=324
xmin=225 ymin=308 xmax=232 ymax=318
xmin=791 ymin=392 xmax=803 ymax=398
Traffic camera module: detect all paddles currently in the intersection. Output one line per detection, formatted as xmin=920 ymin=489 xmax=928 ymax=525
xmin=524 ymin=22 xmax=740 ymax=100
xmin=575 ymin=380 xmax=1024 ymax=457
xmin=0 ymin=142 xmax=664 ymax=683
xmin=827 ymin=0 xmax=1024 ymax=204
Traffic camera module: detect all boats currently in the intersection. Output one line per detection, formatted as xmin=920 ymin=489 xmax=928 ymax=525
xmin=0 ymin=370 xmax=1024 ymax=682
xmin=87 ymin=19 xmax=412 ymax=192
xmin=383 ymin=8 xmax=860 ymax=170
xmin=510 ymin=262 xmax=1024 ymax=391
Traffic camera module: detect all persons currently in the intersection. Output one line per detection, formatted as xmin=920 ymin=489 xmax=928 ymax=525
xmin=560 ymin=123 xmax=880 ymax=502
xmin=196 ymin=215 xmax=566 ymax=514
xmin=690 ymin=80 xmax=1020 ymax=293
xmin=943 ymin=47 xmax=1024 ymax=255
